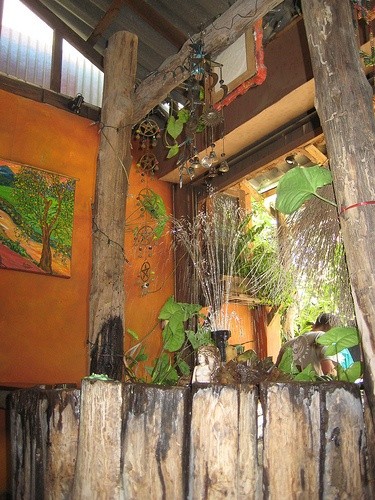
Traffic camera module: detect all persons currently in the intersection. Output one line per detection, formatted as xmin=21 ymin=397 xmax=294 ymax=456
xmin=330 ymin=344 xmax=355 ymax=372
xmin=272 ymin=311 xmax=340 ymax=378
